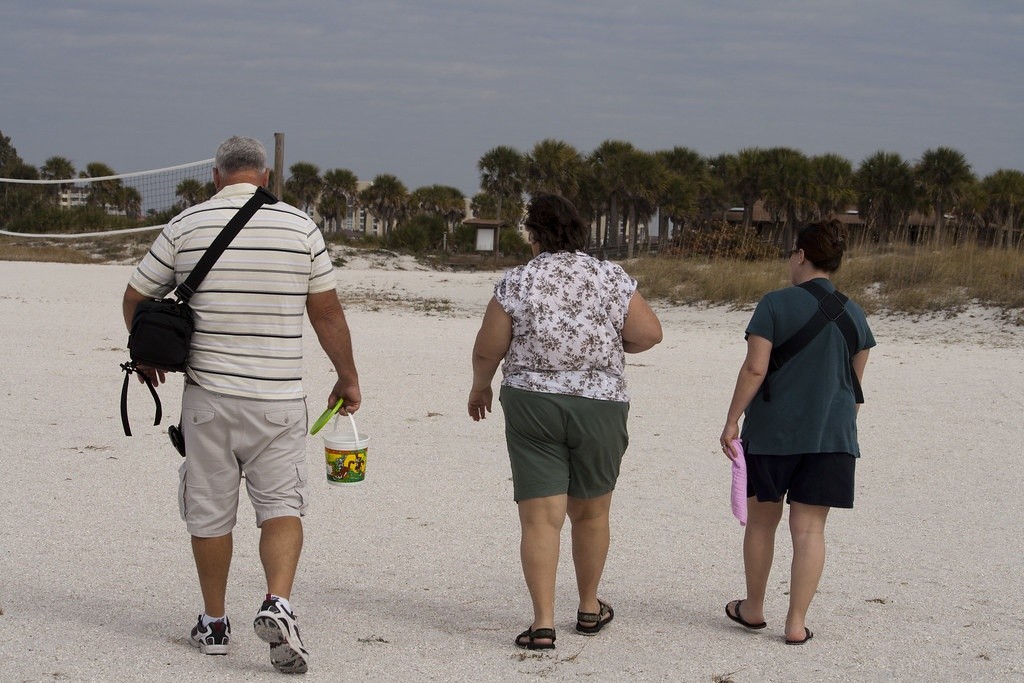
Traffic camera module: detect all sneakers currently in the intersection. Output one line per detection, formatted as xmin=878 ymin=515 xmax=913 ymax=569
xmin=254 ymin=593 xmax=309 ymax=674
xmin=189 ymin=613 xmax=231 ymax=655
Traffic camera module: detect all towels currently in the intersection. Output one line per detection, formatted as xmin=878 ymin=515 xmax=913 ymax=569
xmin=727 ymin=439 xmax=747 ymax=526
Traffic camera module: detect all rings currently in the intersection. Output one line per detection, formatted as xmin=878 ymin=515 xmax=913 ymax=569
xmin=722 ymin=444 xmax=727 ymax=448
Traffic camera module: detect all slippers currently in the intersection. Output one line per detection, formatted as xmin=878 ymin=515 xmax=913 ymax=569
xmin=725 ymin=600 xmax=767 ymax=629
xmin=786 ymin=627 xmax=814 ymax=645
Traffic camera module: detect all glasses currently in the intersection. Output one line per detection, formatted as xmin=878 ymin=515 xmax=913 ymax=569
xmin=787 ymin=249 xmax=803 ymax=259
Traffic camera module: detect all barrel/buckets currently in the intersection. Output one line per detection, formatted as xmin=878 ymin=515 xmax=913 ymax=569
xmin=323 ymin=412 xmax=370 ymax=486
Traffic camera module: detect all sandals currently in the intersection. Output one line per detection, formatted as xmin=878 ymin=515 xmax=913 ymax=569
xmin=515 ymin=625 xmax=556 ymax=650
xmin=575 ymin=599 xmax=614 ymax=636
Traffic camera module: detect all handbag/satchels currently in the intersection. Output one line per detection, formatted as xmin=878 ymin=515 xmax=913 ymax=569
xmin=126 ymin=298 xmax=193 ymax=373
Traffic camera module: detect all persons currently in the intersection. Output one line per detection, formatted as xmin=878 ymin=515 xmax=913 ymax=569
xmin=468 ymin=194 xmax=663 ymax=648
xmin=122 ymin=137 xmax=362 ymax=673
xmin=719 ymin=222 xmax=878 ymax=643
